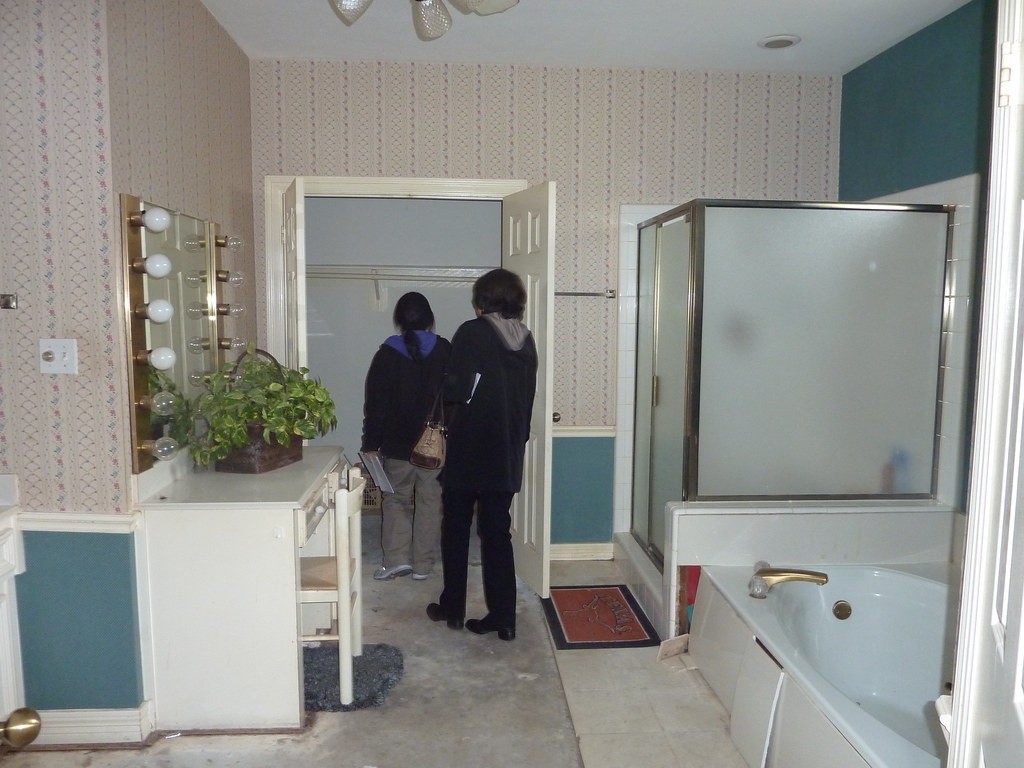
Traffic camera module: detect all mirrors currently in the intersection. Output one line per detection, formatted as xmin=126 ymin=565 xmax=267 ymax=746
xmin=119 ymin=193 xmax=216 ymax=474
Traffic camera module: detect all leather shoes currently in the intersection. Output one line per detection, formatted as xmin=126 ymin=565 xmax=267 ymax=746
xmin=426 ymin=603 xmax=464 ymax=629
xmin=465 ymin=619 xmax=515 ymax=641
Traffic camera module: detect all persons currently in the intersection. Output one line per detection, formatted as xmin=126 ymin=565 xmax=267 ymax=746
xmin=360 ymin=292 xmax=452 ymax=581
xmin=425 ymin=269 xmax=539 ymax=639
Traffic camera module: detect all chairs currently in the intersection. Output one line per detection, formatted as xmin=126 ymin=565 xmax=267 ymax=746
xmin=294 ymin=468 xmax=365 ymax=707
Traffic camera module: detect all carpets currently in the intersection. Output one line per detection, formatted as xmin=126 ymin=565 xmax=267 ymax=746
xmin=301 ymin=641 xmax=404 ymax=712
xmin=542 ymin=583 xmax=662 ymax=650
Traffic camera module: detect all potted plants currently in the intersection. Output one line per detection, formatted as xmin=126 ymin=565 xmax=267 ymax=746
xmin=185 ymin=360 xmax=338 ymax=477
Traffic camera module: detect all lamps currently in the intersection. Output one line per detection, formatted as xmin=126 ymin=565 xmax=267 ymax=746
xmin=329 ymin=0 xmax=519 ymax=42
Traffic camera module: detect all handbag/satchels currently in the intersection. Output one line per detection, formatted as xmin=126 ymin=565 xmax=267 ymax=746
xmin=411 ymin=392 xmax=448 ymax=469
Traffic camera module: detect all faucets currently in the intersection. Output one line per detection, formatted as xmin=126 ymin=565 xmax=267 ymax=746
xmin=751 ymin=568 xmax=829 ymax=592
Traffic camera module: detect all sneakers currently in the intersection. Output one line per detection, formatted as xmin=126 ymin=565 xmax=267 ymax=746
xmin=374 ymin=563 xmax=428 ymax=580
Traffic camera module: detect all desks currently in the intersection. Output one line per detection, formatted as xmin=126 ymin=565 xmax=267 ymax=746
xmin=134 ymin=442 xmax=347 ymax=739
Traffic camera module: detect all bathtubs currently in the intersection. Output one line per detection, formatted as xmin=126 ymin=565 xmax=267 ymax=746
xmin=687 ymin=564 xmax=958 ymax=768
